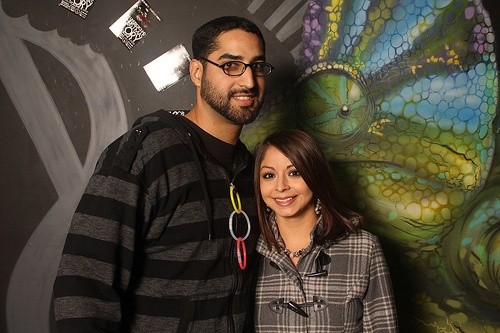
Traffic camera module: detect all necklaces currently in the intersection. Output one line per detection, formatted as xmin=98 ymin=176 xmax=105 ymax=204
xmin=269 ymin=211 xmax=321 ymax=256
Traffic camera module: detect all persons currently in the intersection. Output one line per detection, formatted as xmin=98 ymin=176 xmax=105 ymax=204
xmin=50 ymin=16 xmax=274 ymax=333
xmin=251 ymin=130 xmax=399 ymax=333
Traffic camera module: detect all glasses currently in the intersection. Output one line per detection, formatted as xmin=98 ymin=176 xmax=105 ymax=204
xmin=200 ymin=56 xmax=274 ymax=76
